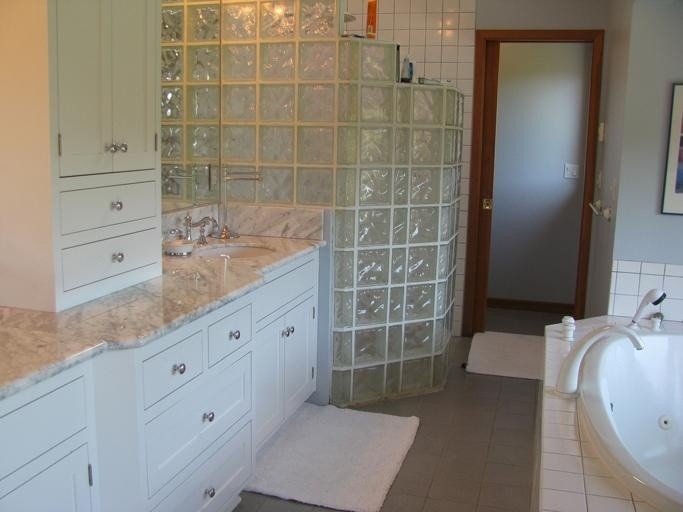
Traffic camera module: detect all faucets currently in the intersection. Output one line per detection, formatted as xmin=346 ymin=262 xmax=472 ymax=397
xmin=556 ymin=322 xmax=644 ymax=397
xmin=182 ymin=214 xmax=219 ymax=240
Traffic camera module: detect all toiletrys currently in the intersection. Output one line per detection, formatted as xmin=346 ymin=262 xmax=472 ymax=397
xmin=400 ymin=55 xmax=410 ymax=83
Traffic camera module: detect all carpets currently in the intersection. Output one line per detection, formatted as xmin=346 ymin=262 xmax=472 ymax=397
xmin=466 ymin=330 xmax=544 ymax=381
xmin=244 ymin=402 xmax=420 ymax=512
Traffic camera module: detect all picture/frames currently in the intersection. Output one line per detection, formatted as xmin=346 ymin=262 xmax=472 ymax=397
xmin=660 ymin=82 xmax=683 ymax=215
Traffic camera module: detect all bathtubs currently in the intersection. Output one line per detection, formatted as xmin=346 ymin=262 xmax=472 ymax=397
xmin=577 ymin=322 xmax=683 ymax=511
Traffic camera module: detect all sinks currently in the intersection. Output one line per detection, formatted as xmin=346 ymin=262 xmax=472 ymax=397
xmin=192 ymin=241 xmax=278 ymax=259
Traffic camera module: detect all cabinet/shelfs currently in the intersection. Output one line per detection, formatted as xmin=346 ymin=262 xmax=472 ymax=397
xmin=135 ymin=290 xmax=254 ymax=512
xmin=254 ymin=247 xmax=318 ymax=454
xmin=49 ymin=1 xmax=162 ymax=314
xmin=0 ymin=353 xmax=100 ymax=511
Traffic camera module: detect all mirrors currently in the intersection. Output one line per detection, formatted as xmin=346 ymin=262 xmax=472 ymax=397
xmin=162 ymin=2 xmax=221 ymax=214
